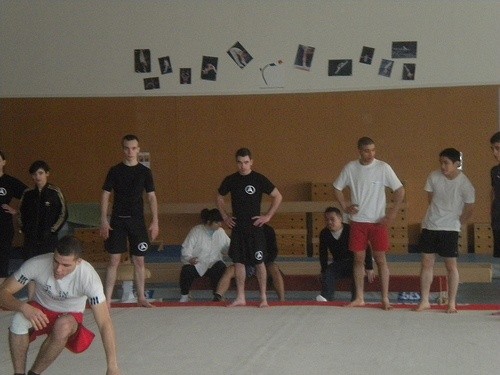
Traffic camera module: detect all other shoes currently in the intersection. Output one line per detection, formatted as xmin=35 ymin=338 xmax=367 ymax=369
xmin=179 ymin=295 xmax=190 ymax=302
xmin=316 ymin=294 xmax=327 ymax=301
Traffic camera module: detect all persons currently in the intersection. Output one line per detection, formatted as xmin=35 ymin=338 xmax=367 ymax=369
xmin=99 ymin=135 xmax=160 ymax=307
xmin=18 ymin=160 xmax=69 ymax=302
xmin=0 ymin=152 xmax=26 ymax=286
xmin=0 ymin=235 xmax=119 ymax=375
xmin=316 ymin=207 xmax=376 ymax=303
xmin=413 ymin=148 xmax=476 ymax=314
xmin=489 ymin=132 xmax=500 ymax=318
xmin=332 ymin=137 xmax=404 ymax=311
xmin=180 ymin=148 xmax=287 ymax=309
